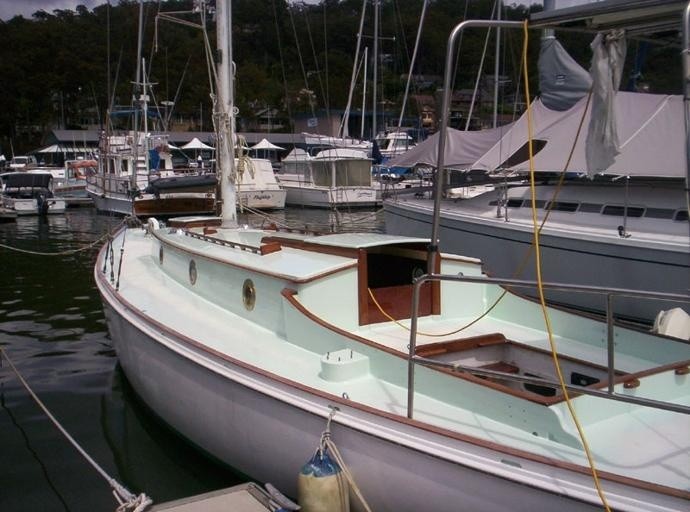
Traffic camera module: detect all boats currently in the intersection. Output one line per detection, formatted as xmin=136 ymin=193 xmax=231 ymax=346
xmin=87 ymin=0 xmax=689 ymax=512
xmin=376 ymin=0 xmax=690 ymax=340
